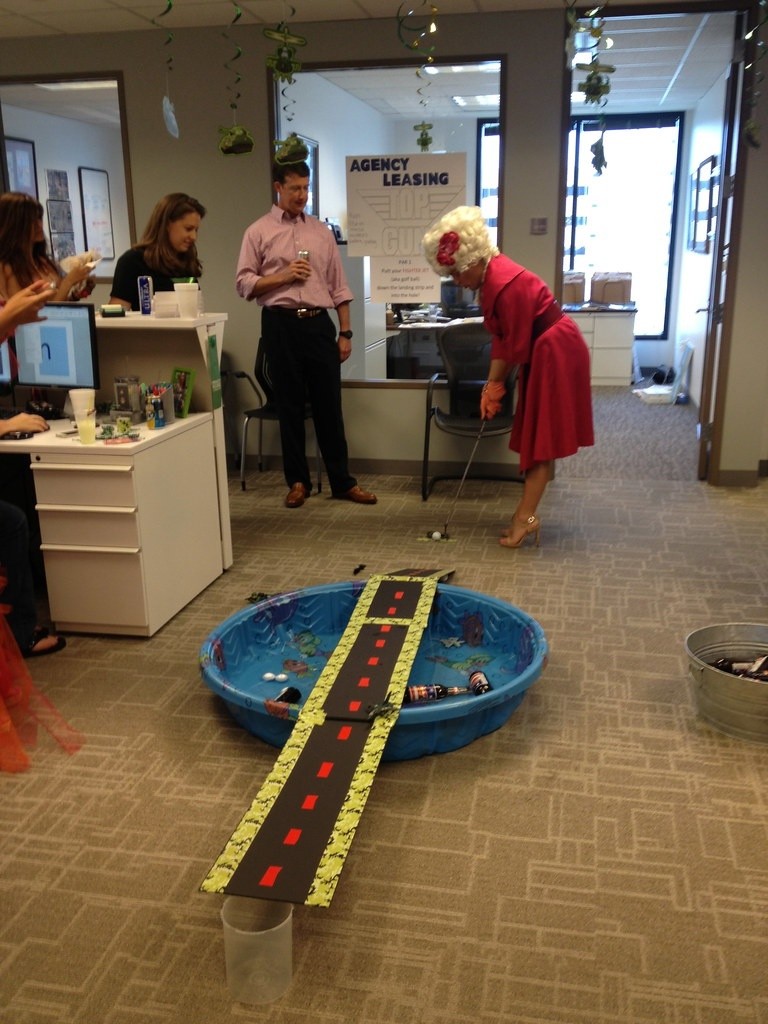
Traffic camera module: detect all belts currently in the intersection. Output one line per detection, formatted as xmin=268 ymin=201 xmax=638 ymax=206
xmin=266 ymin=306 xmax=323 ymax=320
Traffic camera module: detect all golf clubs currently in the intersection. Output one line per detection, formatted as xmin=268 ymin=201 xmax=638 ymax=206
xmin=426 ymin=408 xmax=488 ymax=540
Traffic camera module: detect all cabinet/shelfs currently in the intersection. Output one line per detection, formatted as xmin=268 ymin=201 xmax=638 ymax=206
xmin=561 ymin=301 xmax=635 ymax=387
xmin=0 ymin=311 xmax=233 ymax=638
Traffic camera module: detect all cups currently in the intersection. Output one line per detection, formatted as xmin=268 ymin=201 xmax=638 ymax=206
xmin=386 ymin=312 xmax=394 ymax=325
xmin=428 ymin=304 xmax=438 ymax=323
xmin=69 ymin=388 xmax=95 ymax=409
xmin=174 ymin=283 xmax=199 ymax=320
xmin=112 ymin=375 xmax=139 ymax=410
xmin=73 ymin=408 xmax=96 ymax=444
xmin=220 ymin=895 xmax=297 ymax=1006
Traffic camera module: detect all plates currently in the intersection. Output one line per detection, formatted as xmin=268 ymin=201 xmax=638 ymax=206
xmin=153 ymin=290 xmax=203 ymax=317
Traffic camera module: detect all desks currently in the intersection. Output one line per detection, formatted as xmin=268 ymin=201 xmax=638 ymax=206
xmin=386 ymin=320 xmax=448 ymax=379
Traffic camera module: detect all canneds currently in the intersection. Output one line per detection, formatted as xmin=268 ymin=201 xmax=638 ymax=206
xmin=138 ymin=275 xmax=154 ymax=316
xmin=145 ymin=397 xmax=166 ymax=429
xmin=297 ymin=250 xmax=310 ymax=278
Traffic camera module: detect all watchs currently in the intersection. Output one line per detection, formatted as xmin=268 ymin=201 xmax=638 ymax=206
xmin=339 ymin=330 xmax=353 ymax=340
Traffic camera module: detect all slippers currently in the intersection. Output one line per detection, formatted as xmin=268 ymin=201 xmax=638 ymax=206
xmin=21 ymin=625 xmax=66 ymax=660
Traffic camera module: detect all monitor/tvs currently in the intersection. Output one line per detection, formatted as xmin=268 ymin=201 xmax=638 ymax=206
xmin=0 ymin=302 xmax=101 ymax=390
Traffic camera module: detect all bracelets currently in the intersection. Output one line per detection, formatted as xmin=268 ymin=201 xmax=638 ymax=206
xmin=482 ymin=379 xmax=503 ymax=396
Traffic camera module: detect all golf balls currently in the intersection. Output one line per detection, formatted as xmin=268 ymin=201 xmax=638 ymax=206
xmin=431 ymin=531 xmax=441 ymax=541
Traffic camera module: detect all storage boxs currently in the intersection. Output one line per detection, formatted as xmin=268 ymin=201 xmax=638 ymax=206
xmin=562 ymin=272 xmax=584 ymax=303
xmin=591 ymin=272 xmax=631 ymax=305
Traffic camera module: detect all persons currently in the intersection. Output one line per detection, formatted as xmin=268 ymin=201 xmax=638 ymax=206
xmin=0 ymin=191 xmax=98 ymax=302
xmin=422 ymin=203 xmax=594 ymax=552
xmin=109 ymin=192 xmax=208 ymax=318
xmin=0 ymin=278 xmax=93 ymax=776
xmin=236 ymin=161 xmax=377 ymax=508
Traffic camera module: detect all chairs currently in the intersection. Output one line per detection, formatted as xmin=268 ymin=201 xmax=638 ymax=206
xmin=421 ymin=321 xmax=521 ymax=500
xmin=232 ymin=338 xmax=322 ymax=495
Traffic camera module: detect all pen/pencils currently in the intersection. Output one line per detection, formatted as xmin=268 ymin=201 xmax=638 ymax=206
xmin=147 ymin=387 xmax=149 ymax=396
xmin=149 ymin=381 xmax=170 ymax=396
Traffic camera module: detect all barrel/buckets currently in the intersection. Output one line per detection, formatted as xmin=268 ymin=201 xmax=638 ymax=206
xmin=684 ymin=621 xmax=768 ymax=742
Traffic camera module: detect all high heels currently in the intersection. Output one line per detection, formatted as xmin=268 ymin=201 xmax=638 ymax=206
xmin=499 ymin=509 xmax=542 ymax=549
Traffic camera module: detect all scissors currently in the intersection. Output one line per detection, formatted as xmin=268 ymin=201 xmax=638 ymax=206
xmin=138 ymin=383 xmax=147 ymax=395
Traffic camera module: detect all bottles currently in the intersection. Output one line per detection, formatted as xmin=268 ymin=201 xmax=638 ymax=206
xmin=469 ymin=670 xmax=490 ymax=695
xmin=273 ymin=686 xmax=302 ymax=703
xmin=402 ymin=684 xmax=470 ymax=705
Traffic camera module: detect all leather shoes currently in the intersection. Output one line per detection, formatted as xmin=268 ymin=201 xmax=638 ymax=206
xmin=286 ymin=481 xmax=310 ymax=508
xmin=334 ymin=484 xmax=378 ymax=505
